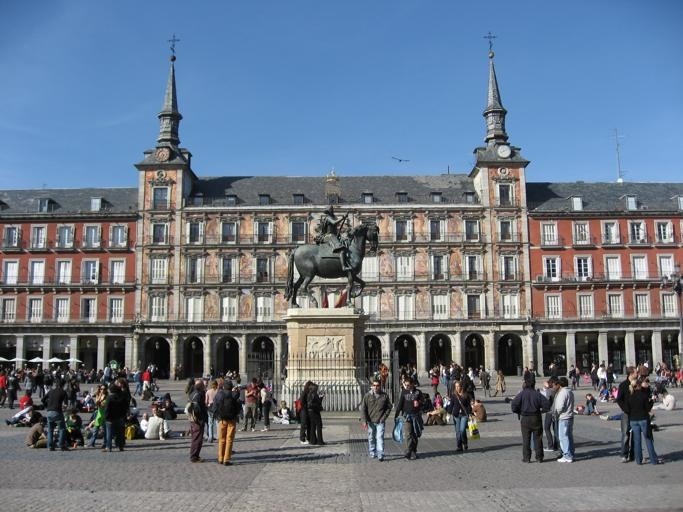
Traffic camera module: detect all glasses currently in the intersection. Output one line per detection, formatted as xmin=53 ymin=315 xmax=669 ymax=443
xmin=100 ymin=389 xmax=106 ymax=390
xmin=405 ymin=384 xmax=410 ymax=386
xmin=373 ymin=385 xmax=380 ymax=386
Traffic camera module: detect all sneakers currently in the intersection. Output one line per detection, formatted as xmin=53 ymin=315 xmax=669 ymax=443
xmin=191 ymin=458 xmax=203 ymax=463
xmin=224 ymin=462 xmax=233 ymax=466
xmin=620 ymin=458 xmax=627 ymax=463
xmin=238 ymin=428 xmax=247 ymax=431
xmin=521 ymin=459 xmax=530 ymax=463
xmin=198 ymin=457 xmax=204 ymax=462
xmin=464 ymin=443 xmax=468 ymax=449
xmin=218 ymin=461 xmax=223 ymax=464
xmin=537 ymin=459 xmax=543 ymax=463
xmin=250 ymin=427 xmax=255 ymax=432
xmin=316 ymin=440 xmax=326 ymax=445
xmin=101 ymin=449 xmax=110 ymax=452
xmin=299 ymin=437 xmax=309 ymax=444
xmin=456 ymin=447 xmax=463 ymax=450
xmin=410 ymin=451 xmax=417 ymax=460
xmin=378 ymin=455 xmax=383 ymax=461
xmin=370 ymin=452 xmax=375 ymax=459
xmin=310 ymin=441 xmax=316 ymax=445
xmin=543 ymin=447 xmax=555 ymax=451
xmin=654 ymin=460 xmax=664 ymax=464
xmin=557 ymin=457 xmax=573 ymax=463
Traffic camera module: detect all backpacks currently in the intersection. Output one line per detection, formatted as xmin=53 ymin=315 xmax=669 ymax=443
xmin=218 ymin=390 xmax=236 ymax=419
xmin=184 ymin=393 xmax=202 ymax=422
xmin=414 ymin=389 xmax=434 ymax=413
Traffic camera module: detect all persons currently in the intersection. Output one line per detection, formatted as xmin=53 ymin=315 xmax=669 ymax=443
xmin=209 ymin=380 xmax=241 ymax=467
xmin=371 ymin=356 xmax=682 ymax=452
xmin=616 ymin=365 xmax=640 ymax=463
xmin=510 ymin=377 xmax=550 ymax=464
xmin=184 ymin=379 xmax=208 ymax=466
xmin=550 ymin=378 xmax=564 ymax=459
xmin=624 ymin=375 xmax=665 ymax=465
xmin=2 ymin=363 xmax=327 ymax=450
xmin=394 ymin=376 xmax=427 ymax=461
xmin=359 ymin=377 xmax=394 ymax=461
xmin=554 ymin=376 xmax=576 ymax=464
xmin=314 ymin=203 xmax=354 ymax=274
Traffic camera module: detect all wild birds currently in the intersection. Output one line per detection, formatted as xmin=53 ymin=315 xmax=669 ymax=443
xmin=392 ymin=156 xmax=410 ymax=162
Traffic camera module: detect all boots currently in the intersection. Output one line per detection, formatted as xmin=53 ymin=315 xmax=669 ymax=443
xmin=261 ymin=425 xmax=270 ymax=432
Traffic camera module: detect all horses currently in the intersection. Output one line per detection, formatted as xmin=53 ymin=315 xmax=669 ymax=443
xmin=283 ymin=223 xmax=380 ymax=309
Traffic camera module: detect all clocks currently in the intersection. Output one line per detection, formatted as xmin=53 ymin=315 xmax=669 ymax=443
xmin=154 ymin=148 xmax=171 ymax=163
xmin=496 ymin=145 xmax=512 ymax=159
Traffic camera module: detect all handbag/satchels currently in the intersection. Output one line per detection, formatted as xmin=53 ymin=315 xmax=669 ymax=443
xmin=295 ymin=400 xmax=302 ymax=413
xmin=127 ymin=426 xmax=134 ymax=440
xmin=64 ymin=389 xmax=74 ymax=399
xmin=392 ymin=416 xmax=404 ymax=442
xmin=467 ymin=416 xmax=480 ymax=440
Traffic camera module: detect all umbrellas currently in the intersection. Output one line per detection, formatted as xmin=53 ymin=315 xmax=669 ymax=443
xmin=0 ymin=355 xmax=86 ymax=364
